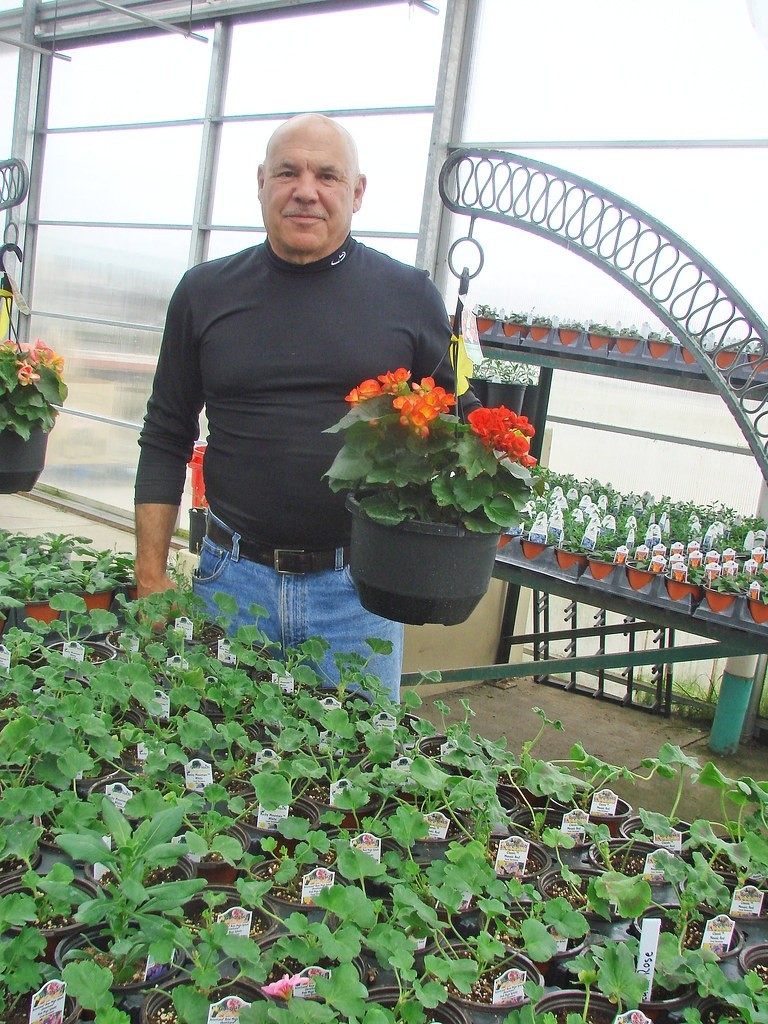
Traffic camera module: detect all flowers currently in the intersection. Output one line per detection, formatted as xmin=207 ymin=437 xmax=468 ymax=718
xmin=0 ymin=336 xmax=70 ymax=441
xmin=319 ymin=367 xmax=538 ymax=533
xmin=261 ymin=972 xmax=309 ymax=1003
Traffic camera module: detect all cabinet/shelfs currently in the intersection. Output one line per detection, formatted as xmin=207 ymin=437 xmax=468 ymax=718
xmin=480 ymin=343 xmax=768 ymax=686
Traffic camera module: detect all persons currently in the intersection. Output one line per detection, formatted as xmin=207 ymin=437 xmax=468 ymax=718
xmin=134 ymin=114 xmax=484 ymax=709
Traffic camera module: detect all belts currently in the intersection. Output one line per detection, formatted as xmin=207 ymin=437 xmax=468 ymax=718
xmin=205 ymin=517 xmax=351 ymax=576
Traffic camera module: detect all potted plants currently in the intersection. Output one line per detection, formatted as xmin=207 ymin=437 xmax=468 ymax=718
xmin=0 ymin=528 xmax=768 ymax=1024
xmin=454 ymin=304 xmax=768 ymax=374
xmin=495 ymin=463 xmax=768 ymax=624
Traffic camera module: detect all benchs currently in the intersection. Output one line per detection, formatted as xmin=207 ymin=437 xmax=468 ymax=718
xmin=52 ymin=329 xmax=159 ymax=394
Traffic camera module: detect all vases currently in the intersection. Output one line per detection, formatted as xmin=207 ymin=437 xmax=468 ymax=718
xmin=344 ymin=491 xmax=504 ymax=627
xmin=0 ymin=426 xmax=50 ymax=495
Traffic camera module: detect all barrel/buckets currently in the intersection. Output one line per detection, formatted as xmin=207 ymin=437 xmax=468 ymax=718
xmin=188 ymin=445 xmax=209 ymax=508
